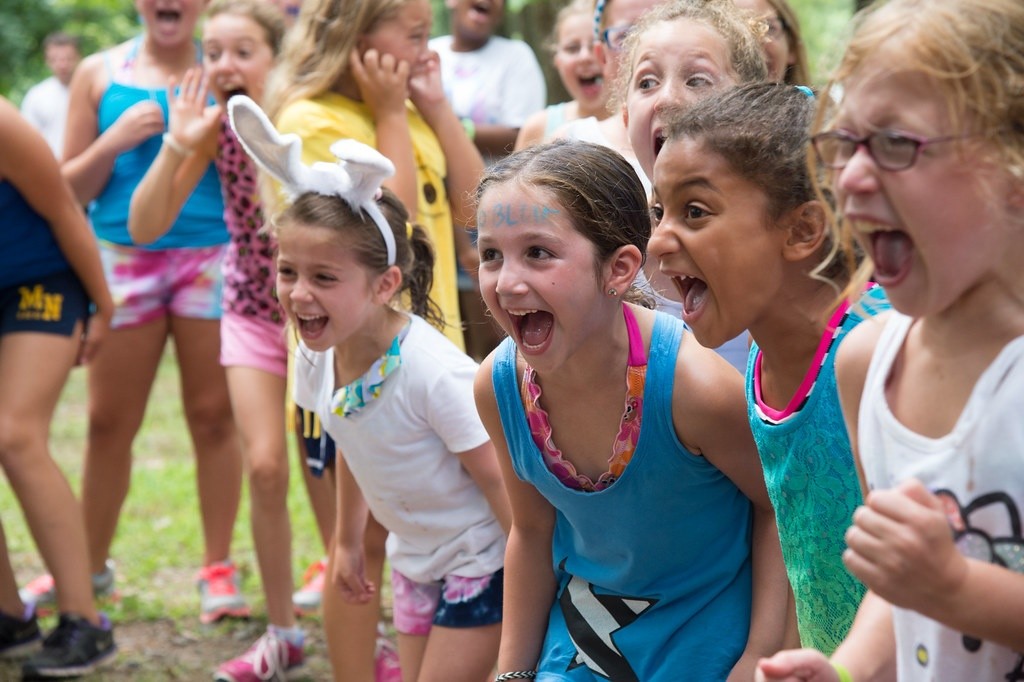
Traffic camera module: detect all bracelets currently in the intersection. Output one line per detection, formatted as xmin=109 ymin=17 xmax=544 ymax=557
xmin=828 ymin=660 xmax=851 ymax=682
xmin=496 ymin=670 xmax=537 ymax=682
xmin=161 ymin=133 xmax=195 ymax=159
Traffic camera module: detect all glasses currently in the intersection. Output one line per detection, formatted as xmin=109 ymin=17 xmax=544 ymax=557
xmin=806 ymin=129 xmax=976 ymax=173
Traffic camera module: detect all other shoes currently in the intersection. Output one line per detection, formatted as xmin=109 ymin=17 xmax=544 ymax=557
xmin=18 ymin=563 xmax=118 ymax=616
xmin=195 ymin=558 xmax=251 ymax=624
xmin=288 ymin=556 xmax=330 ymax=618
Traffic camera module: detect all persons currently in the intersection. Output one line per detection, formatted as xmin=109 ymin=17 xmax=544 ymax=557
xmin=471 ymin=137 xmax=800 ymax=681
xmin=647 ymin=83 xmax=894 ymax=658
xmin=1 ymin=0 xmax=1024 ymax=682
xmin=268 ymin=183 xmax=515 ymax=681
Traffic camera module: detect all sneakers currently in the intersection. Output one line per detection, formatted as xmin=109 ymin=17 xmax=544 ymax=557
xmin=1 ymin=603 xmax=44 ymax=659
xmin=212 ymin=624 xmax=309 ymax=682
xmin=370 ymin=621 xmax=404 ymax=682
xmin=21 ymin=612 xmax=118 ymax=679
